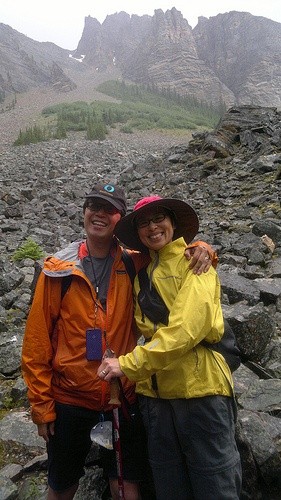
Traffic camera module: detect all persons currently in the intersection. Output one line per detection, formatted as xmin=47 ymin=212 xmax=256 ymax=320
xmin=21 ymin=180 xmax=218 ymax=500
xmin=96 ymin=196 xmax=243 ymax=500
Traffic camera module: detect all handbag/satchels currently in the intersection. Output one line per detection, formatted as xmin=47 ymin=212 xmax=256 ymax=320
xmin=222 ymin=317 xmax=242 ymax=373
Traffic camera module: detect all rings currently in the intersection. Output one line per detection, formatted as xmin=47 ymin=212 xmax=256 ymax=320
xmin=204 ymin=256 xmax=209 ymax=261
xmin=101 ymin=371 xmax=106 ymax=375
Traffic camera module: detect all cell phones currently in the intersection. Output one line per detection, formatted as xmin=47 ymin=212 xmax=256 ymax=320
xmin=86 ymin=328 xmax=102 ymax=360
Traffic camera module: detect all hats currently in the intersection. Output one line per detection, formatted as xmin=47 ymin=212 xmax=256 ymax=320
xmin=115 ymin=195 xmax=204 ymax=241
xmin=81 ymin=177 xmax=126 ymax=216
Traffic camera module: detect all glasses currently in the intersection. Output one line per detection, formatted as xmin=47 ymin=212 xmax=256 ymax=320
xmin=85 ymin=201 xmax=117 ymax=215
xmin=138 ymin=213 xmax=166 ymax=228
